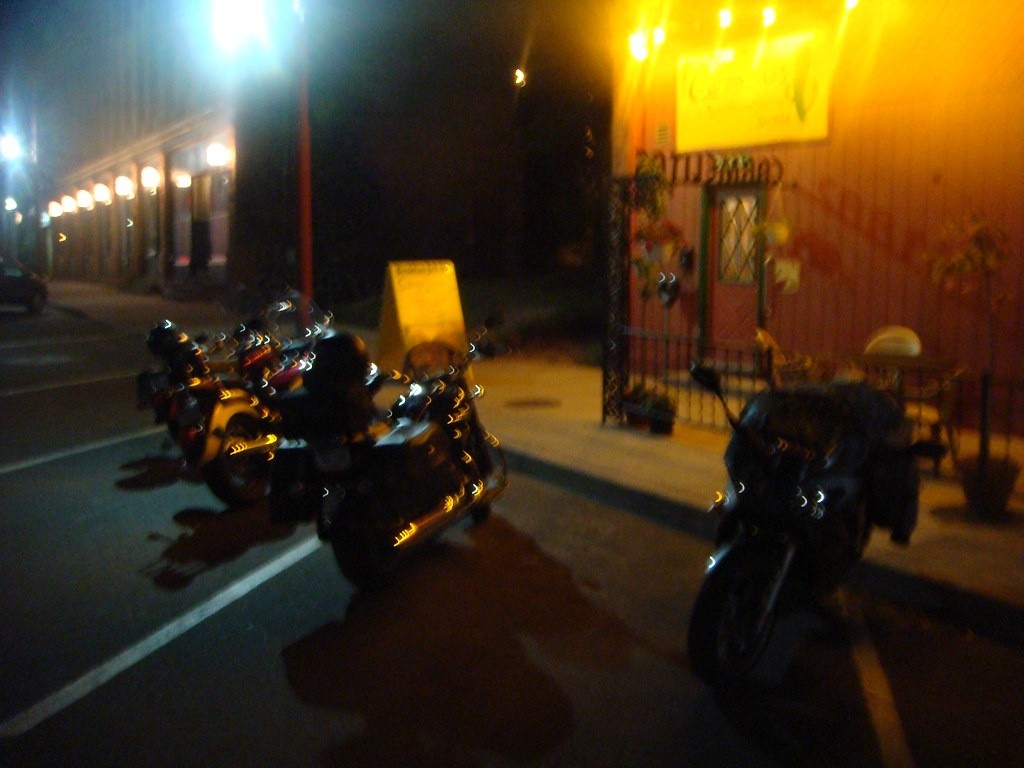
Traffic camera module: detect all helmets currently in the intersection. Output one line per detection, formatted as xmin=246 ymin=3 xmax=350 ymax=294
xmin=301 ymin=330 xmax=371 ymax=401
xmin=145 ymin=320 xmax=180 ymax=361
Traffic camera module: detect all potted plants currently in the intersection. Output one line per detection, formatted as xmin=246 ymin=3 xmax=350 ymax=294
xmin=953 ymin=226 xmax=1023 ymax=520
xmin=620 ymin=155 xmax=686 ymax=434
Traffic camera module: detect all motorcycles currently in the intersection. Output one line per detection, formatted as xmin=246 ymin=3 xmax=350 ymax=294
xmin=135 ymin=291 xmax=511 ymax=593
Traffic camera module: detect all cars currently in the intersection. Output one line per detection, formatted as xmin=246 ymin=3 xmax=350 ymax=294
xmin=0 ymin=251 xmax=50 ymax=313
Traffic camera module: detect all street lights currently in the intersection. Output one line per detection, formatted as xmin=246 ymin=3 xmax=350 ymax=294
xmin=198 ymin=1 xmax=312 ymax=333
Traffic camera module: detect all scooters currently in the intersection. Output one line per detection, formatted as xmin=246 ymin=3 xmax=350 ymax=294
xmin=683 ymin=322 xmax=952 ymax=690
